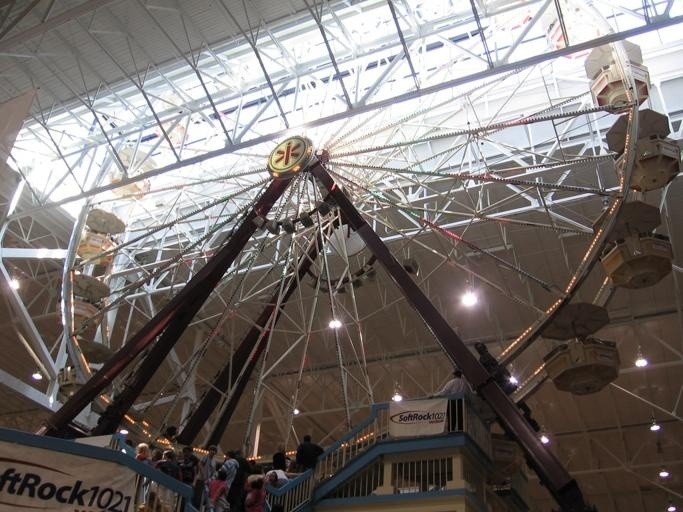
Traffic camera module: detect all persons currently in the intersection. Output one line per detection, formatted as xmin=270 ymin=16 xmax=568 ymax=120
xmin=123 ymin=424 xmax=327 ymax=512
xmin=426 ymin=369 xmax=473 ymax=432
